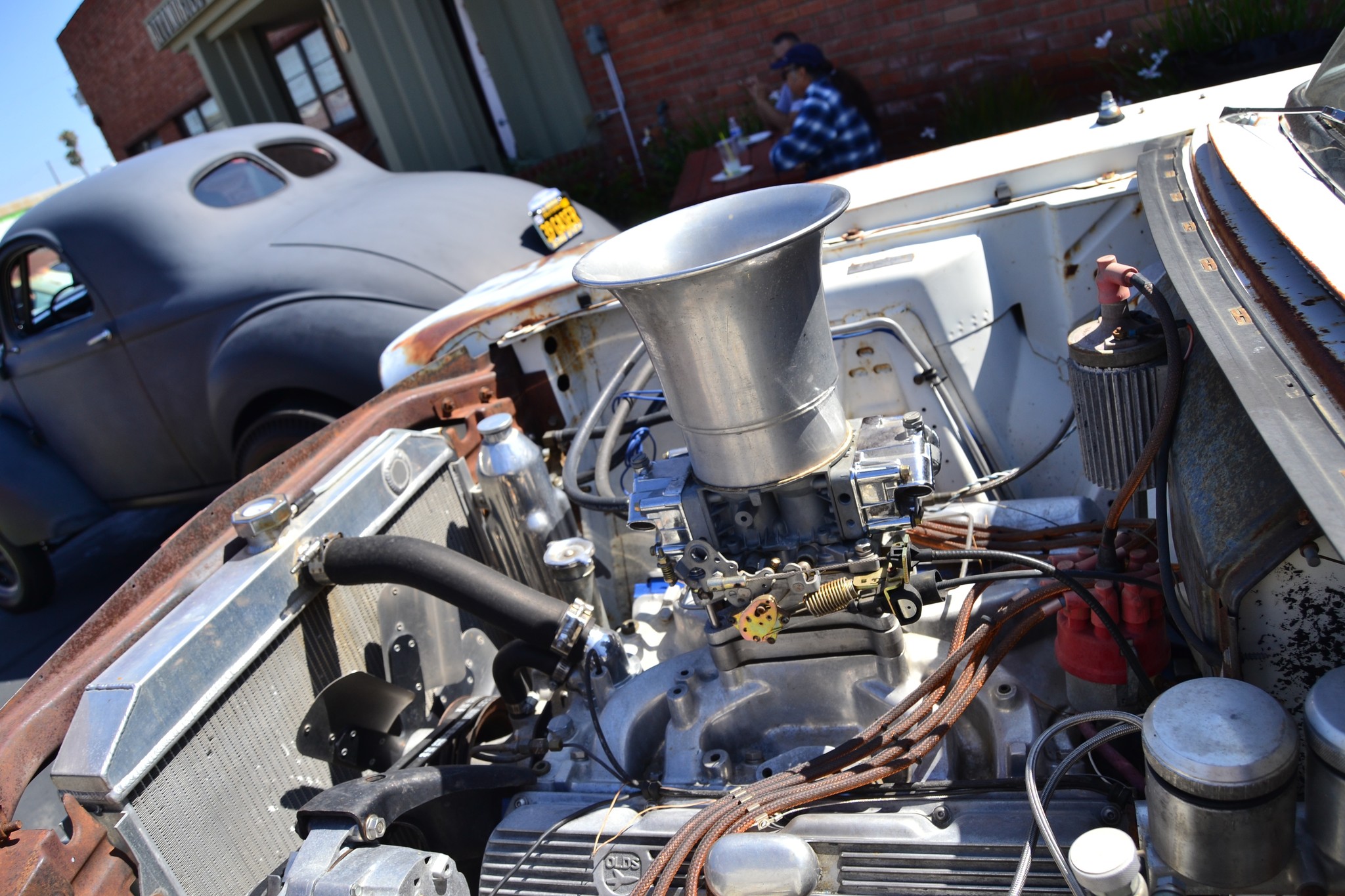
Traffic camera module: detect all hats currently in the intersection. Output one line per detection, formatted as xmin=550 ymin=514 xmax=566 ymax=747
xmin=768 ymin=41 xmax=823 ymax=69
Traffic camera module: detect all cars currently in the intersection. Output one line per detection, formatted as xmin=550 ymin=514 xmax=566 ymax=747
xmin=1 ymin=120 xmax=631 ymax=614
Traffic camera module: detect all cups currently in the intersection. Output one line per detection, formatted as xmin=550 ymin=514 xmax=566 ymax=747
xmin=716 ymin=137 xmax=742 ymax=178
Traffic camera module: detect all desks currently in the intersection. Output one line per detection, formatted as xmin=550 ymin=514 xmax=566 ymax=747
xmin=670 ymin=134 xmax=805 ymax=212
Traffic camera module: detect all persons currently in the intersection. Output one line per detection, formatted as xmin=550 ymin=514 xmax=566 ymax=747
xmin=769 ymin=42 xmax=889 ymax=178
xmin=745 ymin=32 xmax=802 ymax=135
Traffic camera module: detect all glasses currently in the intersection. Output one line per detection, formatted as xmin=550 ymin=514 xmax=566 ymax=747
xmin=780 ymin=64 xmax=800 ymax=81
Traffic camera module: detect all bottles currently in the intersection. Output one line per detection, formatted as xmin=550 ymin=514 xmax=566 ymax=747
xmin=728 ymin=116 xmax=748 ymax=152
